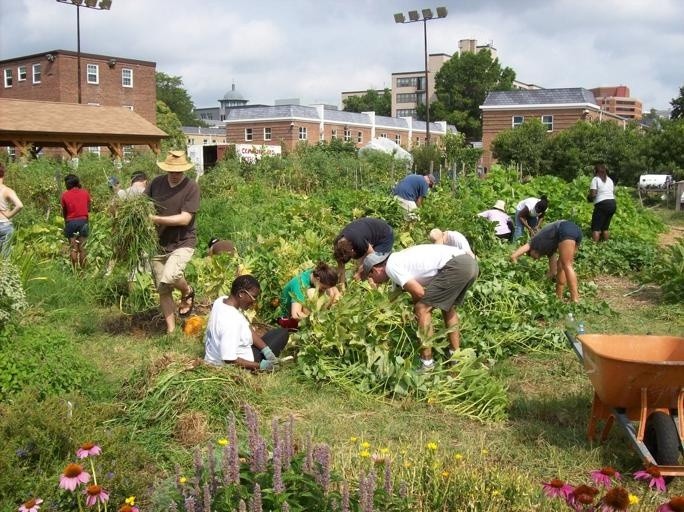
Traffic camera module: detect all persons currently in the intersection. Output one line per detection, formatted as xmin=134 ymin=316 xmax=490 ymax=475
xmin=333 ymin=218 xmax=393 ymax=281
xmin=393 ymin=174 xmax=438 ymax=221
xmin=587 ymin=164 xmax=617 ymax=242
xmin=364 ymin=244 xmax=478 ymax=369
xmin=112 ymin=172 xmax=148 ymax=292
xmin=514 ymin=195 xmax=549 ymax=239
xmin=60 ymin=174 xmax=91 ymax=268
xmin=143 ymin=150 xmax=199 ymax=335
xmin=430 ymin=229 xmax=475 ymax=260
xmin=208 ymin=237 xmax=234 ymax=255
xmin=283 ymin=261 xmax=341 ymax=323
xmin=205 ymin=275 xmax=289 ymax=374
xmin=476 ymin=200 xmax=515 ymax=242
xmin=510 ymin=219 xmax=583 ymax=304
xmin=0 ymin=163 xmax=24 ymax=256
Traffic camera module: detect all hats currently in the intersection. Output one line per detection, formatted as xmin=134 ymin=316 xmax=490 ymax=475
xmin=428 ymin=174 xmax=436 ymax=189
xmin=208 ymin=237 xmax=219 ymax=248
xmin=363 ymin=251 xmax=391 ymax=276
xmin=429 ymin=229 xmax=443 ymax=245
xmin=155 ymin=150 xmax=195 ymax=172
xmin=493 ymin=200 xmax=506 ymax=212
xmin=107 ymin=177 xmax=119 ymax=187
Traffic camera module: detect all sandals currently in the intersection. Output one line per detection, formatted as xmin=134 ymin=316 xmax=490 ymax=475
xmin=178 ymin=285 xmax=195 ymax=317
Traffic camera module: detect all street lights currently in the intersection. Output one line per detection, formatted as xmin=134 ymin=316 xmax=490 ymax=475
xmin=57 ymin=1 xmax=112 ymax=104
xmin=393 ymin=6 xmax=449 ymax=148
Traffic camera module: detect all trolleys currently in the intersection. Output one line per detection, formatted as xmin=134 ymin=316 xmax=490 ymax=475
xmin=561 ymin=329 xmax=683 ymax=479
xmin=638 ymin=175 xmax=683 ymax=211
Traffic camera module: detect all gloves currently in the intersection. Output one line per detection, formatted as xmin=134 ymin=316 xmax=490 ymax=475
xmin=260 ymin=360 xmax=279 ymax=372
xmin=260 ymin=347 xmax=276 ymax=360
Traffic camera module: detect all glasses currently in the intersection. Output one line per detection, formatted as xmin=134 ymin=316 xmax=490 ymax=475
xmin=244 ymin=290 xmax=257 ymax=304
